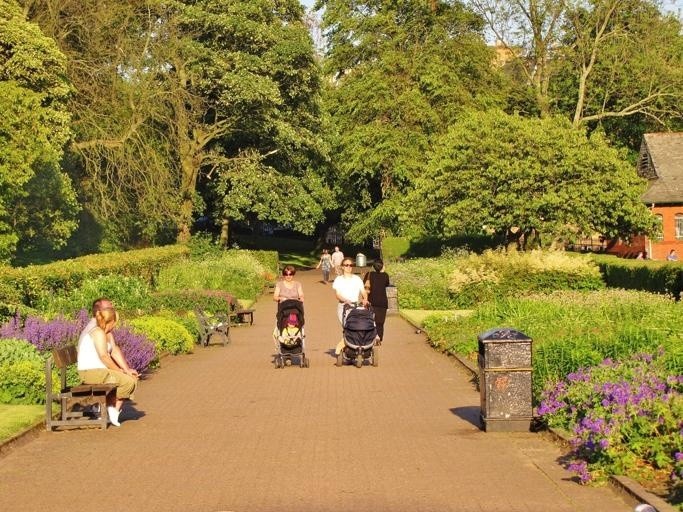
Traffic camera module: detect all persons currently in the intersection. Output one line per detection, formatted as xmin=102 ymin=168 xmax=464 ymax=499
xmin=331 ymin=246 xmax=344 ymax=277
xmin=78 ymin=297 xmax=140 ymax=419
xmin=272 ymin=264 xmax=304 ymax=303
xmin=76 ymin=308 xmax=137 ymax=427
xmin=363 ymin=258 xmax=389 ymax=345
xmin=331 ymin=258 xmax=369 ymax=329
xmin=315 ymin=249 xmax=334 ymax=284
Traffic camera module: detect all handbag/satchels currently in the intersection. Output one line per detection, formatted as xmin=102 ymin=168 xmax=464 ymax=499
xmin=364 ymin=271 xmax=370 ymax=294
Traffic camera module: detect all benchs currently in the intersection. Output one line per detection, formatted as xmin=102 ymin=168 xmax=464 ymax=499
xmin=228 ymin=296 xmax=256 ymax=327
xmin=193 ymin=304 xmax=229 ymax=348
xmin=45 ymin=342 xmax=130 ymax=432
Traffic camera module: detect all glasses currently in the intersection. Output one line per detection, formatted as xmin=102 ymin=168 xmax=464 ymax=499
xmin=285 ymin=273 xmax=291 ymax=276
xmin=343 ymin=264 xmax=352 ymax=267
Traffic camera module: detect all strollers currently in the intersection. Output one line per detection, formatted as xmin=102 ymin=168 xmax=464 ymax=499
xmin=272 ymin=298 xmax=309 ymax=369
xmin=336 ymin=302 xmax=380 ymax=367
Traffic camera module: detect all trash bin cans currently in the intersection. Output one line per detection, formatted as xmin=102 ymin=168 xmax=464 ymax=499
xmin=477 ymin=328 xmax=534 ymax=432
xmin=385 ymin=285 xmax=399 ymax=313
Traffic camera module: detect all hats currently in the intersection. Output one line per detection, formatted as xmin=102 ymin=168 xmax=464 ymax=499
xmin=285 ymin=314 xmax=298 ymax=324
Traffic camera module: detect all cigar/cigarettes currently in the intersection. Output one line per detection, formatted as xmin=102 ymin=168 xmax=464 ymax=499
xmin=138 ymin=373 xmax=143 ymax=378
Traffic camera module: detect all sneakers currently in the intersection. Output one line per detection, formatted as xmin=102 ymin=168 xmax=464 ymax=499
xmin=107 ymin=407 xmax=120 ymax=427
xmin=92 ymin=403 xmax=110 ymax=424
